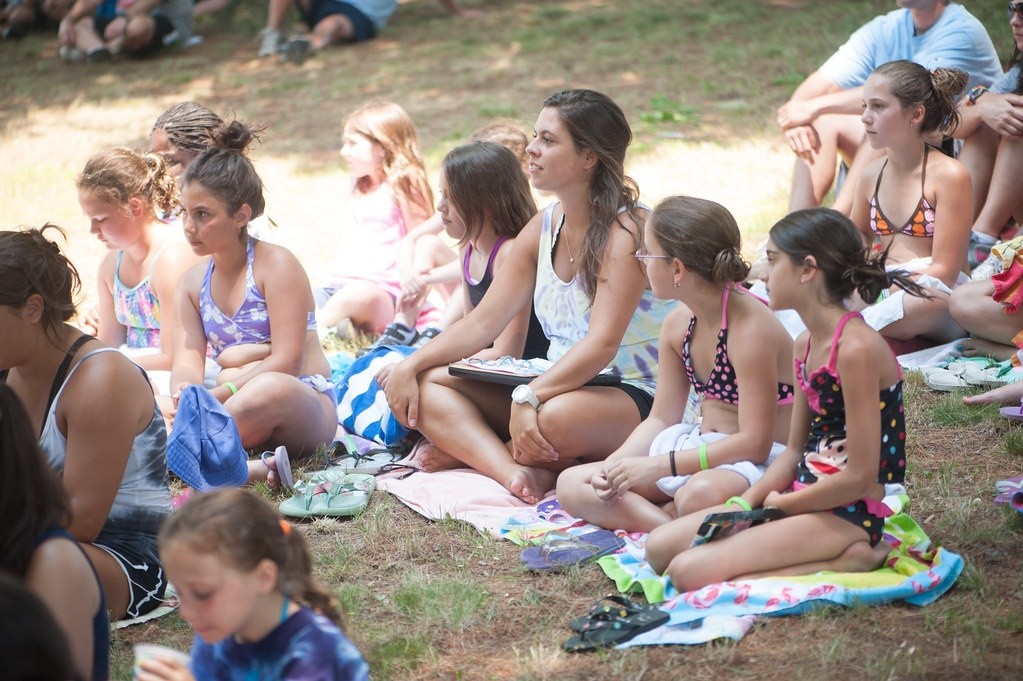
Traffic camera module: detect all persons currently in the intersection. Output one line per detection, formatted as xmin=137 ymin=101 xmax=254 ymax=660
xmin=750 ymin=0 xmax=1023 ymax=405
xmin=0 ymin=95 xmax=907 ymax=681
xmin=0 ymin=0 xmax=483 ymax=63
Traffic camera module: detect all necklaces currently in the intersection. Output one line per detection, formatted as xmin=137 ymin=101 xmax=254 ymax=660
xmin=563 ymin=216 xmax=578 ymax=263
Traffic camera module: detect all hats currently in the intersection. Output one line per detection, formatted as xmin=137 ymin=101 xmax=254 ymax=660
xmin=168 ymin=384 xmax=250 ymax=493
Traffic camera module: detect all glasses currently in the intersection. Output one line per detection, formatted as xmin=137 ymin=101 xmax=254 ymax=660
xmin=634 ymin=248 xmax=673 ymax=261
xmin=1007 ymin=1 xmax=1023 ymax=18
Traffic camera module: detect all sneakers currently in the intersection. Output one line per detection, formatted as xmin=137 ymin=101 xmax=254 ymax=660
xmin=408 ymin=325 xmax=440 ymax=349
xmin=356 ymin=320 xmax=421 ymax=361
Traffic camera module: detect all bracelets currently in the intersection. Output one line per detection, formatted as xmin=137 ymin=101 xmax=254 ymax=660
xmin=966 ymin=85 xmax=989 ymax=106
xmin=224 ymin=382 xmax=239 ymax=395
xmin=670 ymin=450 xmax=677 ymax=477
xmin=724 ymin=496 xmax=752 ymax=512
xmin=699 ymin=444 xmax=708 ymax=471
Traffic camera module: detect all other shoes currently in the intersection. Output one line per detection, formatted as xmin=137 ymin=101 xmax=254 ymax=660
xmin=286 ymin=35 xmax=317 ymax=68
xmin=335 ymin=317 xmax=356 ymax=345
xmin=59 ymin=45 xmax=86 ymax=62
xmin=1 ymin=26 xmax=15 ymax=37
xmin=257 ymin=26 xmax=287 ymax=56
xmin=87 ymin=45 xmax=112 ymax=62
xmin=175 ymin=33 xmax=203 ymax=48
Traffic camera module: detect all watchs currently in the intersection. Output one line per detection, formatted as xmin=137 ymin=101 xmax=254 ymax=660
xmin=969 ymin=88 xmax=983 ymax=105
xmin=511 ymin=384 xmax=543 ymax=411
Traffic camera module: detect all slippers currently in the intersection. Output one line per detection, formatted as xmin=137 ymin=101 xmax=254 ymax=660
xmin=278 ymin=469 xmax=376 ymax=517
xmin=324 ymin=448 xmax=403 ymax=475
xmin=922 ymin=355 xmax=1021 ymax=391
xmin=520 ymin=528 xmax=628 ymax=572
xmin=561 ymin=594 xmax=671 ymax=653
xmin=999 ymin=397 xmax=1023 ymax=421
xmin=262 ymin=444 xmax=294 ymax=494
xmin=993 ymin=477 xmax=1022 ymax=503
